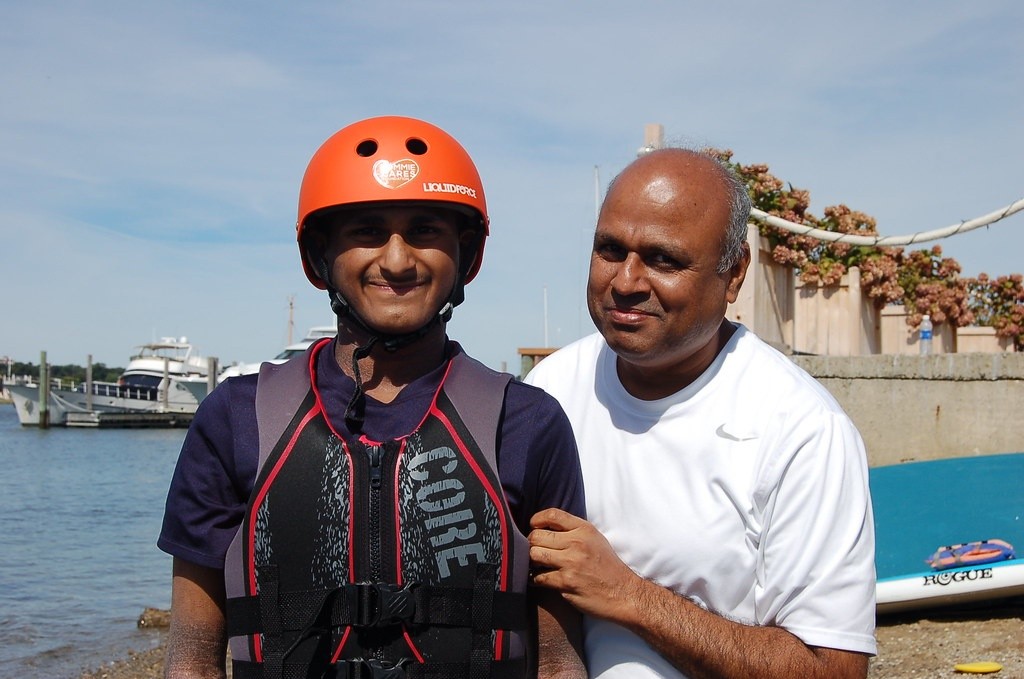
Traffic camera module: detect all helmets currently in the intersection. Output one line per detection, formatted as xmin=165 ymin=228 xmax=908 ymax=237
xmin=296 ymin=115 xmax=490 ymax=290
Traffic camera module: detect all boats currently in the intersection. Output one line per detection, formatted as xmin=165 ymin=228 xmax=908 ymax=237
xmin=10 ymin=390 xmax=65 ymax=427
xmin=117 ymin=335 xmax=229 ymax=400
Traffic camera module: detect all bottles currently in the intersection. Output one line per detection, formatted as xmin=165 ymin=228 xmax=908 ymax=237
xmin=920 ymin=315 xmax=933 ymax=355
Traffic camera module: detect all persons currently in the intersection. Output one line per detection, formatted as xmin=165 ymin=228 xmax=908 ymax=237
xmin=522 ymin=145 xmax=877 ymax=679
xmin=157 ymin=117 xmax=588 ymax=679
